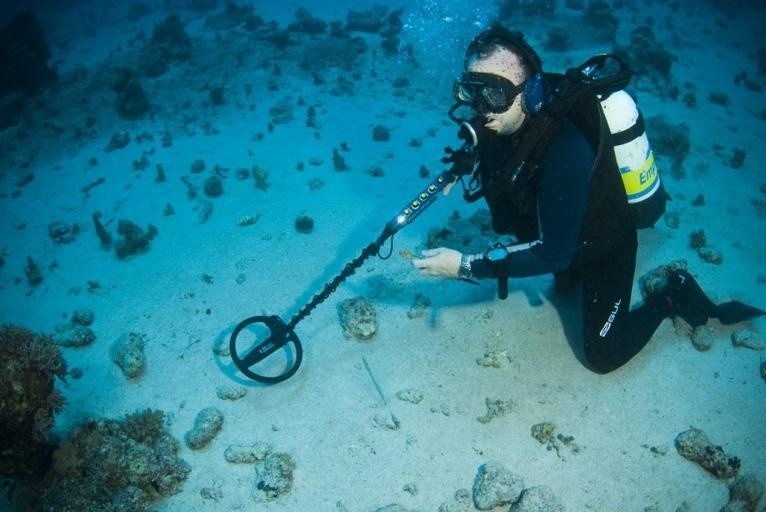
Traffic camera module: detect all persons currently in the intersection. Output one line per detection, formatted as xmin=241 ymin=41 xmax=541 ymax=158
xmin=413 ymin=22 xmax=707 ymax=374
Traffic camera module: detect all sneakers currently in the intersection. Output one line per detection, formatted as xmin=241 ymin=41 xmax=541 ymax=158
xmin=669 ymin=270 xmax=708 ymax=327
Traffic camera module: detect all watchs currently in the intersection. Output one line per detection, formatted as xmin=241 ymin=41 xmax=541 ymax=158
xmin=458 ymin=254 xmax=472 ymax=280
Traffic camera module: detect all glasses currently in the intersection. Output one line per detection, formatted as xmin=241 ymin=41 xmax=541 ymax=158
xmin=453 ymin=71 xmax=517 ymax=114
